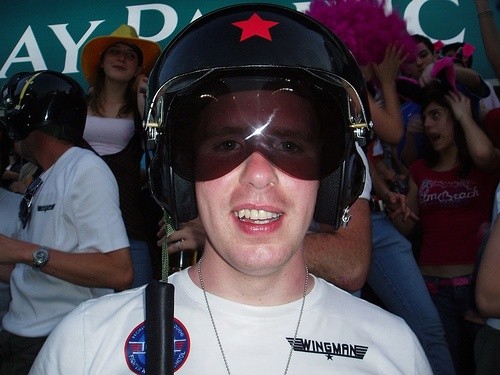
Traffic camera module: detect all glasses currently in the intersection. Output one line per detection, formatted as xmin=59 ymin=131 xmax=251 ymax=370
xmin=17 ymin=178 xmax=43 ymax=230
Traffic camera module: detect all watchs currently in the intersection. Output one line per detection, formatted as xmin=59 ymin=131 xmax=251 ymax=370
xmin=31 ymin=245 xmax=50 ymax=270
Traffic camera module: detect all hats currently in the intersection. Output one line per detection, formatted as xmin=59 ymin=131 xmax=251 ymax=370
xmin=81 ymin=25 xmax=161 ymax=87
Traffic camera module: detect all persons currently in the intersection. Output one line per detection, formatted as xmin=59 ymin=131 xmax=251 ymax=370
xmin=0 ymin=70 xmax=134 ymax=375
xmin=29 ymin=3 xmax=435 ymax=375
xmin=156 ymin=140 xmax=372 ymax=301
xmin=195 ymin=256 xmax=309 ymax=374
xmin=80 ymin=23 xmax=162 ymax=155
xmin=291 ymin=0 xmax=500 ymax=375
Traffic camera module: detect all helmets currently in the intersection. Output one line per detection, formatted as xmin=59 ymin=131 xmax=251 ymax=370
xmin=0 ymin=70 xmax=87 ymax=142
xmin=139 ymin=2 xmax=373 ymax=159
